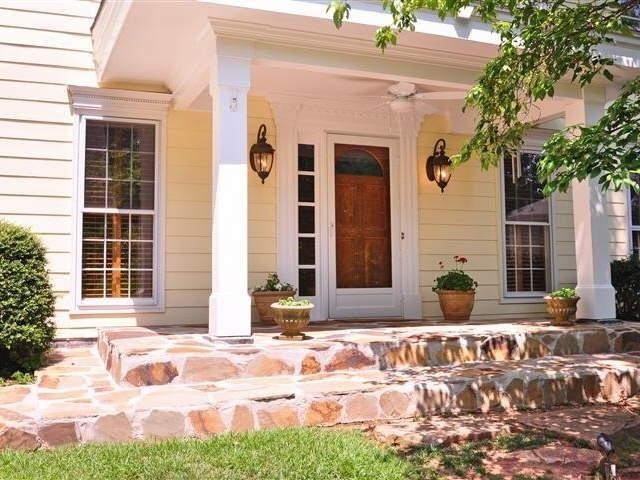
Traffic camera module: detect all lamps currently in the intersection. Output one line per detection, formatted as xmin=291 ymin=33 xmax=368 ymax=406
xmin=249 ymin=124 xmax=276 ymax=184
xmin=425 ymin=138 xmax=451 ymax=194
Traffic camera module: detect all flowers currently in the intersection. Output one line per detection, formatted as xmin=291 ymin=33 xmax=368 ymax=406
xmin=431 ymin=256 xmax=478 ymax=294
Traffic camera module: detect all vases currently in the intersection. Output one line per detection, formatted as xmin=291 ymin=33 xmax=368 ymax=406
xmin=435 ymin=291 xmax=476 ymax=321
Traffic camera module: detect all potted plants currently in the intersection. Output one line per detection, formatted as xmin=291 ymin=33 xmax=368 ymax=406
xmin=268 ymin=296 xmax=315 ymax=340
xmin=543 ymin=288 xmax=582 ymax=326
xmin=251 ymin=272 xmax=299 ymax=326
